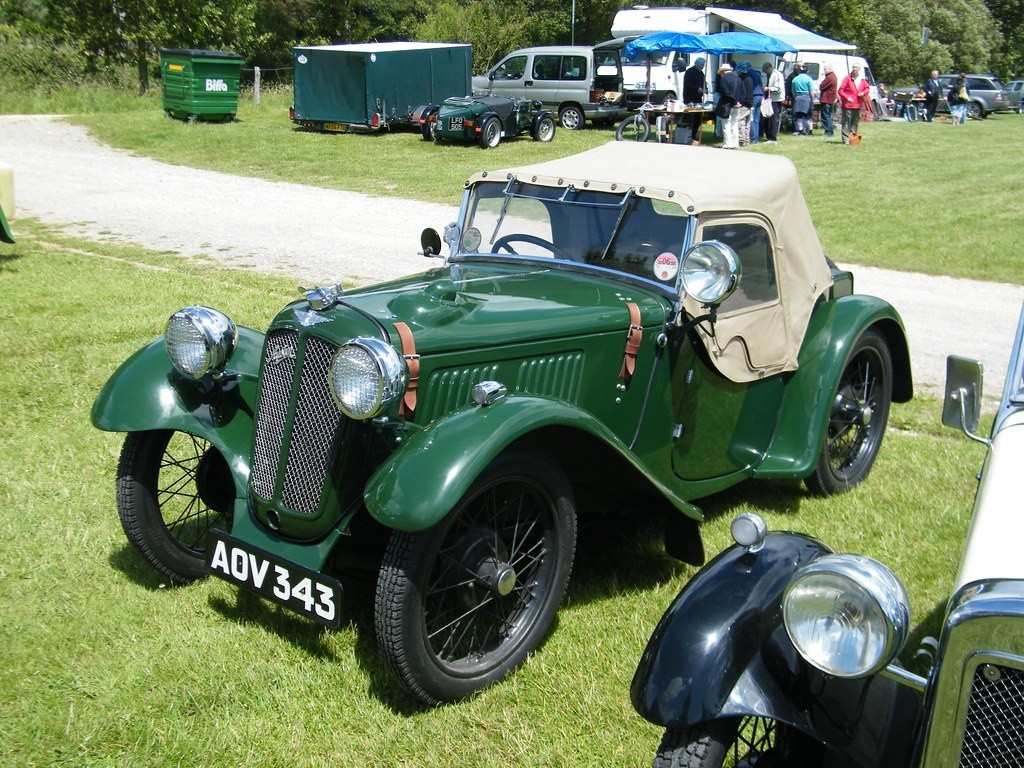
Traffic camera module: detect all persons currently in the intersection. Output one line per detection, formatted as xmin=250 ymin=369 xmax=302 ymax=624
xmin=819 ymin=63 xmax=837 ymax=136
xmin=735 ymin=60 xmax=753 ymax=146
xmin=922 ymin=70 xmax=944 ymax=121
xmin=791 ymin=65 xmax=815 ymax=135
xmin=950 ymin=73 xmax=971 ymax=125
xmin=763 ymin=63 xmax=785 ymax=144
xmin=683 ymin=57 xmax=708 ymax=139
xmin=786 ymin=62 xmax=808 ymax=135
xmin=716 ymin=64 xmax=746 ymax=149
xmin=741 ymin=61 xmax=764 ymax=145
xmin=838 ymin=63 xmax=869 ymax=145
xmin=878 ymin=83 xmax=895 ymax=105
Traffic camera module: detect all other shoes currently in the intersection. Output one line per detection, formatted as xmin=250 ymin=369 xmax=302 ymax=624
xmin=792 ymin=130 xmax=802 ymax=135
xmin=764 ymin=140 xmax=776 ymax=143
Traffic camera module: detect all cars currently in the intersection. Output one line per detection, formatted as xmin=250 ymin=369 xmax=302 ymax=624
xmin=631 ymin=296 xmax=1024 ymax=768
xmin=90 ymin=140 xmax=914 ymax=718
xmin=886 ymin=75 xmax=1009 ymax=119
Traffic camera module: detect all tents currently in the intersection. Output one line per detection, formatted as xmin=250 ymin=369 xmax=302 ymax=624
xmin=621 ymin=31 xmax=798 ymax=131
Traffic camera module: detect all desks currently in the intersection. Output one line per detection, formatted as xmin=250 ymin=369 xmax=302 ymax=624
xmin=893 ymin=97 xmax=951 ymax=116
xmin=632 ymin=108 xmax=715 ymax=145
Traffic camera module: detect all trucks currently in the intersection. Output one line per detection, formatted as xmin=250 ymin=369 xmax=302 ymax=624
xmin=596 ymin=6 xmax=857 ymax=107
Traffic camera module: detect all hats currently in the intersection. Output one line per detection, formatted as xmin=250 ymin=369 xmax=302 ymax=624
xmin=735 ymin=62 xmax=748 ymax=73
xmin=717 ymin=64 xmax=734 ymax=74
xmin=762 ymin=62 xmax=771 ymax=72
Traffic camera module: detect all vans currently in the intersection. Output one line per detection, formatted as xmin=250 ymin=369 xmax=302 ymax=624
xmin=777 ymin=51 xmax=881 ymax=120
xmin=1003 ymin=81 xmax=1024 ymax=114
xmin=473 ymin=35 xmax=642 ymax=128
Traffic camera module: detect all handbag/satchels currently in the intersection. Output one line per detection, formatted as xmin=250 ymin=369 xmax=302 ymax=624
xmin=958 ymin=84 xmax=970 ymax=102
xmin=761 ymin=90 xmax=774 ymax=118
xmin=714 ymin=98 xmax=733 ymax=118
